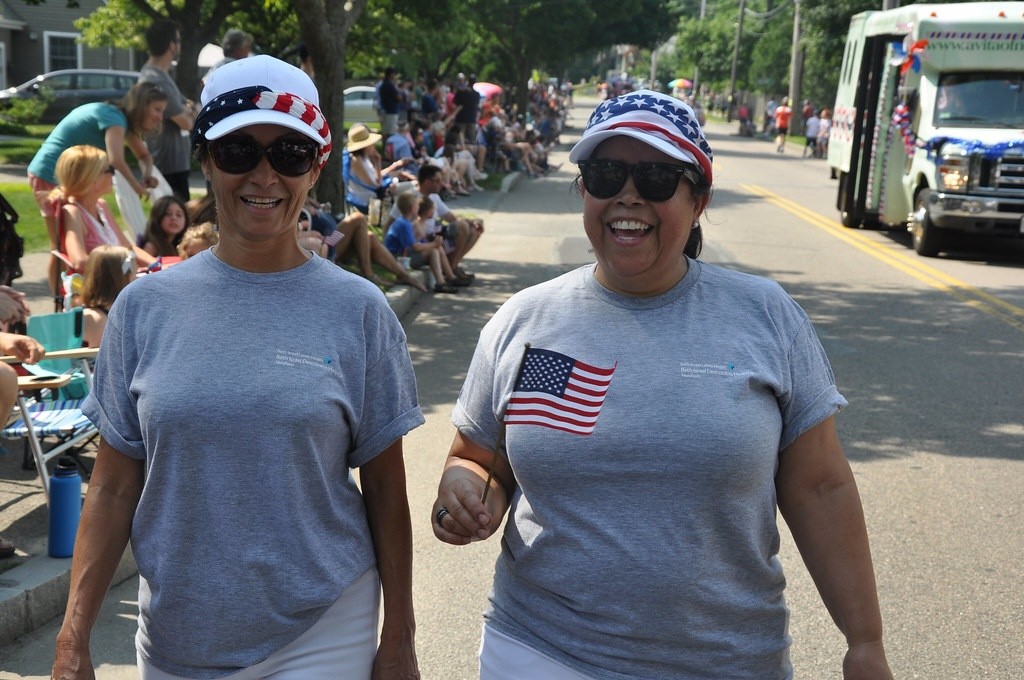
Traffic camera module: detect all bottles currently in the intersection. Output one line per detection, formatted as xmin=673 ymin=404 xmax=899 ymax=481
xmin=48 ymin=458 xmax=81 ymax=558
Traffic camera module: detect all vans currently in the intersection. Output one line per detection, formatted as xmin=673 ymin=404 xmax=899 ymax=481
xmin=0 ymin=69 xmax=139 ymax=122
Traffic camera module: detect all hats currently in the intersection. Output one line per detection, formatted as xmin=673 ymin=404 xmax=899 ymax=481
xmin=570 ymin=89 xmax=714 ymax=187
xmin=396 ymin=119 xmax=409 ymax=129
xmin=342 ymin=123 xmax=379 ymax=154
xmin=191 ymin=52 xmax=332 ymax=173
xmin=347 ymin=125 xmax=382 ymax=152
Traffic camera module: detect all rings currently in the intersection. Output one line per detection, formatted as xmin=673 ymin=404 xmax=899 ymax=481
xmin=436 ymin=507 xmax=449 ymax=526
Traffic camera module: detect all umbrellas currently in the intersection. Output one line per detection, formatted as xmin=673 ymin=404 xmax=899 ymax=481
xmin=667 ymin=79 xmax=692 ymax=87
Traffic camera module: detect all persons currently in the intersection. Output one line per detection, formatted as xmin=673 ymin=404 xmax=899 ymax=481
xmin=597 ymin=76 xmax=835 ymax=159
xmin=0 ymin=21 xmax=571 ymax=556
xmin=46 ymin=54 xmax=420 ymax=680
xmin=432 ymin=89 xmax=894 ymax=680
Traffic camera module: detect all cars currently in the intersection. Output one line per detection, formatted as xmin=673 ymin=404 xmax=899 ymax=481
xmin=343 ymin=85 xmax=379 ymax=121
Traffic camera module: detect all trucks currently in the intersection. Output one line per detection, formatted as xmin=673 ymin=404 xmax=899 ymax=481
xmin=826 ymin=2 xmax=1024 ymax=257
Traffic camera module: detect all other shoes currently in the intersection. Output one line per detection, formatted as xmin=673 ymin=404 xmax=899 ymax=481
xmin=474 ymin=173 xmax=488 ymax=180
xmin=368 ymin=273 xmax=395 ymax=287
xmin=456 ymin=189 xmax=470 ymax=196
xmin=467 ymin=184 xmax=484 ymax=192
xmin=396 ymin=271 xmax=428 ymax=293
xmin=454 ymin=266 xmax=474 ymax=280
xmin=445 ymin=276 xmax=471 ymax=286
xmin=0 ymin=538 xmax=15 ymax=558
xmin=433 ymin=283 xmax=458 ymax=293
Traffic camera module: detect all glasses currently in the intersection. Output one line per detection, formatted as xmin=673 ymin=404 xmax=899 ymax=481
xmin=208 ymin=129 xmax=319 ymax=178
xmin=576 ymin=156 xmax=705 ymax=204
xmin=104 ymin=164 xmax=115 ymax=176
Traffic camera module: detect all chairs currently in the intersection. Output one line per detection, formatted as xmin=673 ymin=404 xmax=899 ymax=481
xmin=0 ymin=197 xmax=100 ymax=509
xmin=342 ymin=152 xmax=391 ymax=216
